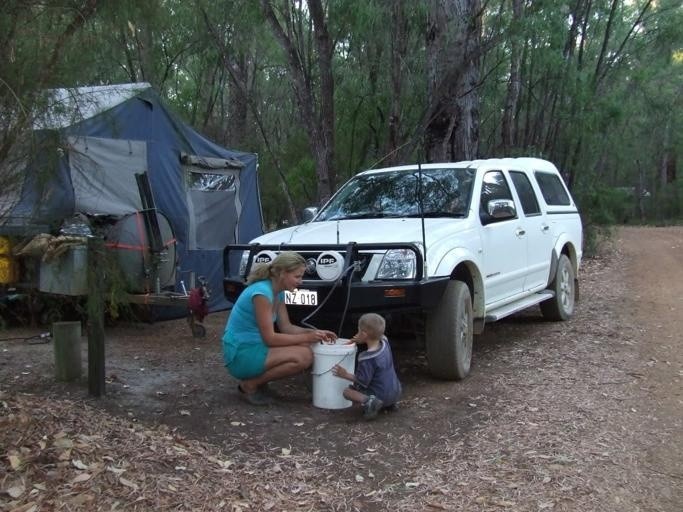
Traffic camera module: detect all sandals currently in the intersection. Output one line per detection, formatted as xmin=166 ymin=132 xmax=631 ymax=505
xmin=234 ymin=382 xmax=277 ymax=406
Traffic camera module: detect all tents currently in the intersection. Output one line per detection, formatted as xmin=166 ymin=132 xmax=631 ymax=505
xmin=2 ymin=82 xmax=266 ymax=325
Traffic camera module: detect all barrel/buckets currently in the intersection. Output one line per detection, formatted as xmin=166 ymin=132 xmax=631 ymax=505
xmin=310 ymin=337 xmax=358 ymax=410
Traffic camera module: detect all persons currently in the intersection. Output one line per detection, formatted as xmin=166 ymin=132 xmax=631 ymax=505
xmin=221 ymin=251 xmax=338 ymax=407
xmin=332 ymin=313 xmax=404 ymax=422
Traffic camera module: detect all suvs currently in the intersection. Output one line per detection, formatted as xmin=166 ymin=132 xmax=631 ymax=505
xmin=224 ymin=158 xmax=584 ymax=379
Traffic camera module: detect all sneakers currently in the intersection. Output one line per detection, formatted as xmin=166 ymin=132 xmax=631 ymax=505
xmin=362 ymin=395 xmax=381 ymax=419
